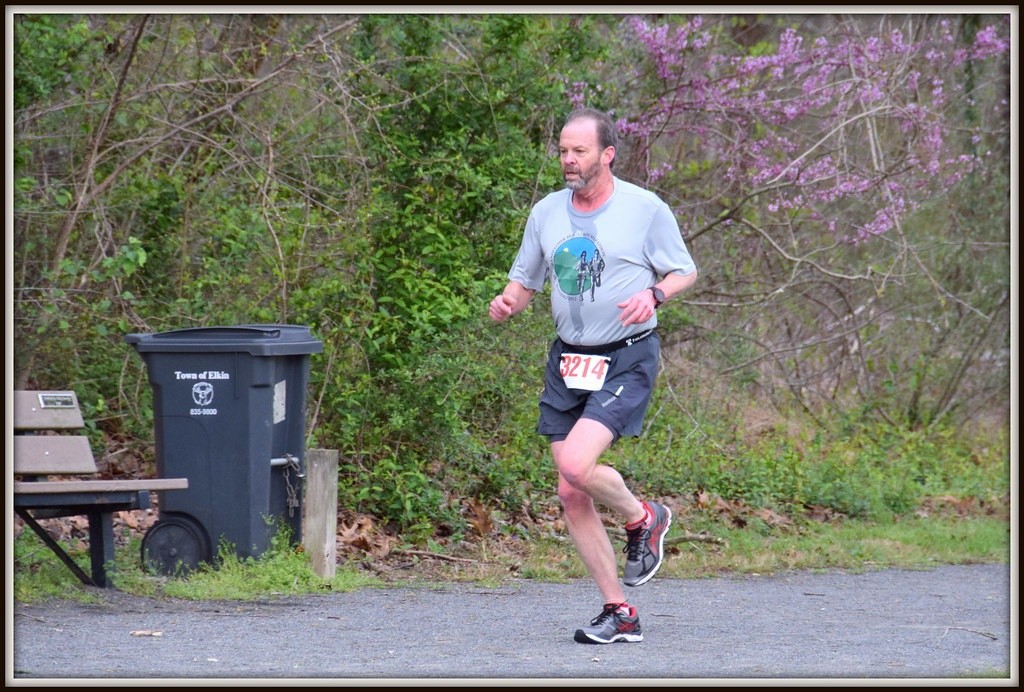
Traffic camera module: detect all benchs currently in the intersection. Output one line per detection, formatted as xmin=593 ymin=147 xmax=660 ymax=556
xmin=13 ymin=389 xmax=190 ymax=588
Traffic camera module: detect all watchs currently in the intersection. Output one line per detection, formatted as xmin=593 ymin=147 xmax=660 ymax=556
xmin=649 ymin=287 xmax=666 ymax=307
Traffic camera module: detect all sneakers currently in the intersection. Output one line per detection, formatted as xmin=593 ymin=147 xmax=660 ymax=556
xmin=623 ymin=501 xmax=672 ymax=587
xmin=573 ymin=599 xmax=643 ymax=644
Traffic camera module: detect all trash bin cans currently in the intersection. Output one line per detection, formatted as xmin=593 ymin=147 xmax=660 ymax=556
xmin=125 ymin=323 xmax=324 ymax=581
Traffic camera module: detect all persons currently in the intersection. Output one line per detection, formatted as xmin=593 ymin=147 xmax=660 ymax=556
xmin=489 ymin=108 xmax=697 ymax=643
